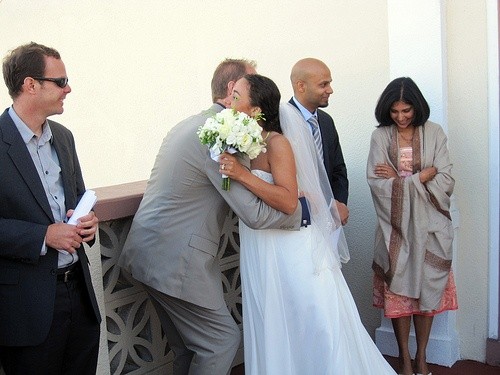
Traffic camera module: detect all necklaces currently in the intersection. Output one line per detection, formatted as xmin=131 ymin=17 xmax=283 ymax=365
xmin=398 ymin=128 xmax=414 ymax=142
xmin=261 ymin=130 xmax=271 ymax=141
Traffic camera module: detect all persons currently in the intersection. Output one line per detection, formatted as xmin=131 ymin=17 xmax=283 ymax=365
xmin=218 ymin=74 xmax=397 ymax=375
xmin=0 ymin=41 xmax=103 ymax=375
xmin=287 ymin=58 xmax=350 ymax=231
xmin=366 ymin=77 xmax=459 ymax=375
xmin=117 ymin=57 xmax=320 ymax=375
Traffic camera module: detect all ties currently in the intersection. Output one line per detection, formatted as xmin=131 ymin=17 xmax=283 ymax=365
xmin=307 ymin=116 xmax=324 ymax=167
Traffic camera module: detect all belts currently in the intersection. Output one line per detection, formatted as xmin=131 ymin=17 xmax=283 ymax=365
xmin=57 ymin=269 xmax=81 ymax=282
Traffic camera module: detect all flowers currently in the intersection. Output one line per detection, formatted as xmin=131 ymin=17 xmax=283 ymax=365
xmin=196 ymin=99 xmax=269 ymax=191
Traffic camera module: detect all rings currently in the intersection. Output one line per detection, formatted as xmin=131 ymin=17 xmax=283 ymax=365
xmin=221 ymin=164 xmax=225 ymax=170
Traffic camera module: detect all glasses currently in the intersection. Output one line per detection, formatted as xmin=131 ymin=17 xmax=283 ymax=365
xmin=23 ymin=76 xmax=68 ymax=88
